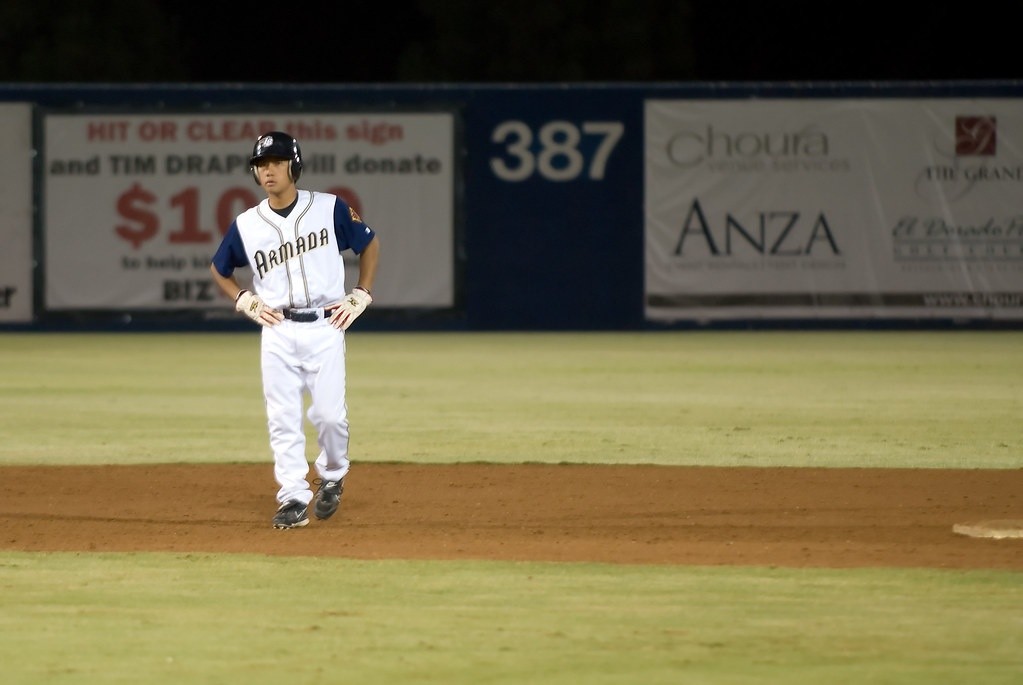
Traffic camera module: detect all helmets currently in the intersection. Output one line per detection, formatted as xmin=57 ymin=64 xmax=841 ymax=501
xmin=249 ymin=132 xmax=302 ymax=185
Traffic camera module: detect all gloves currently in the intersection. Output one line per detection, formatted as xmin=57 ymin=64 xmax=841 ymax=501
xmin=325 ymin=288 xmax=372 ymax=330
xmin=236 ymin=290 xmax=284 ymax=328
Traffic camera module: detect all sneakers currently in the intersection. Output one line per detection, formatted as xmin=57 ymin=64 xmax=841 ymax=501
xmin=272 ymin=499 xmax=310 ymax=530
xmin=313 ymin=478 xmax=345 ymax=519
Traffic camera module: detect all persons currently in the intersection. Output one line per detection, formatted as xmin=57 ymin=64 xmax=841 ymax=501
xmin=210 ymin=132 xmax=379 ymax=527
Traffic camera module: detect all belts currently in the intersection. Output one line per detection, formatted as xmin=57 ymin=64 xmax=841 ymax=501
xmin=283 ymin=308 xmax=332 ymax=322
